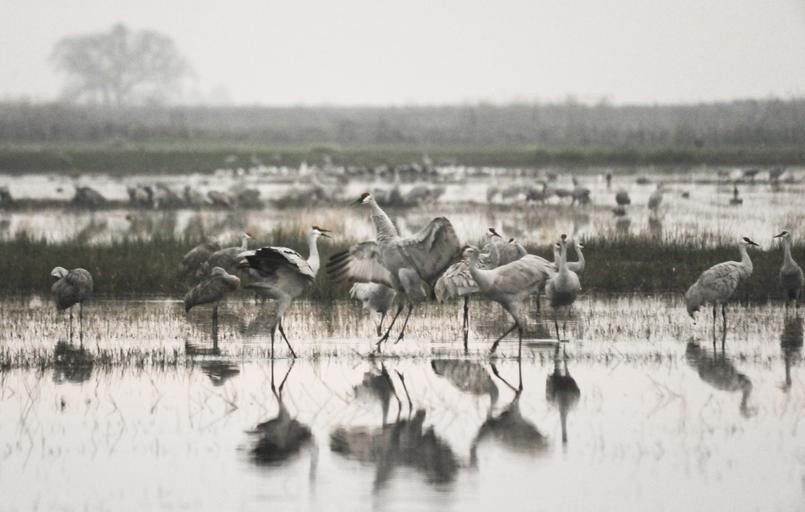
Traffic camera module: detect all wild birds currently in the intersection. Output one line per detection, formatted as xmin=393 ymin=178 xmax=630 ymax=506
xmin=685 ymin=327 xmax=756 ymax=420
xmin=774 ymin=313 xmax=804 ymax=391
xmin=173 ymin=191 xmax=590 ymax=360
xmin=71 ymin=148 xmax=800 ymax=223
xmin=684 ymin=237 xmax=760 ymax=327
xmin=246 ymin=360 xmax=582 ymax=501
xmin=50 ymin=266 xmax=94 ymax=319
xmin=773 ymin=231 xmax=804 ymax=313
